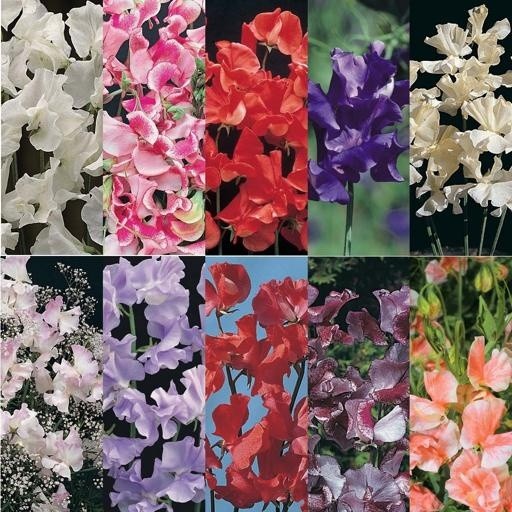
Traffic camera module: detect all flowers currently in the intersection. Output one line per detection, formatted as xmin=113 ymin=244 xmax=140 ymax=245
xmin=2 ymin=0 xmax=100 ymax=255
xmin=1 ymin=255 xmax=100 ymax=511
xmin=206 ymin=0 xmax=309 ymax=260
xmin=411 ymin=257 xmax=511 ymax=512
xmin=310 ymin=38 xmax=409 ymax=257
xmin=408 ymin=4 xmax=511 ymax=255
xmin=204 ymin=263 xmax=306 ymax=512
xmin=306 ymin=284 xmax=409 ymax=512
xmin=102 ymin=1 xmax=204 ymax=257
